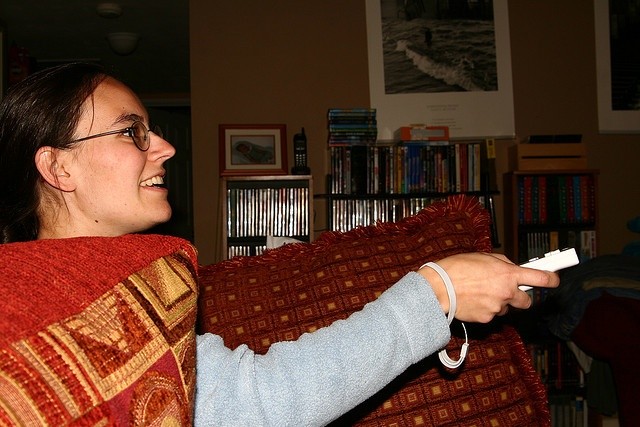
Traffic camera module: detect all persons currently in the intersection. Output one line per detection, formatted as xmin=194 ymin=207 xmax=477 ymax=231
xmin=0 ymin=58 xmax=561 ymax=427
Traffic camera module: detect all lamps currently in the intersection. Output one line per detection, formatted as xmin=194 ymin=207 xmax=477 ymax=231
xmin=107 ymin=31 xmax=140 ymax=55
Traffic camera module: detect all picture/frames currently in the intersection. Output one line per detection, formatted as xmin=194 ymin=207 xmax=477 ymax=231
xmin=594 ymin=1 xmax=640 ymax=137
xmin=136 ymin=91 xmax=193 ymax=243
xmin=218 ymin=123 xmax=289 ymax=177
xmin=366 ymin=0 xmax=516 ymax=141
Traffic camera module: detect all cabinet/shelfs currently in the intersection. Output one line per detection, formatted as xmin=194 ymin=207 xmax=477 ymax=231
xmin=313 ymin=189 xmax=503 ymax=250
xmin=214 ymin=175 xmax=315 ymax=265
xmin=502 ymin=169 xmax=602 ymax=427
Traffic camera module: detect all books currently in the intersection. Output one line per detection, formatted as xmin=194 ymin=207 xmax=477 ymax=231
xmin=332 ymin=196 xmax=487 ymax=233
xmin=524 ymin=288 xmax=549 ymax=305
xmin=519 ymin=230 xmax=599 ymax=258
xmin=518 ymin=173 xmax=593 ymax=225
xmin=525 ymin=342 xmax=563 ymax=390
xmin=330 ymin=144 xmax=487 ymax=194
xmin=508 ymin=130 xmax=596 ymax=172
xmin=394 ymin=124 xmax=450 ymax=145
xmin=229 ymin=243 xmax=267 ymax=258
xmin=548 ymin=396 xmax=591 ymax=427
xmin=227 ymin=186 xmax=309 ymax=239
xmin=326 ymin=109 xmax=377 ymax=144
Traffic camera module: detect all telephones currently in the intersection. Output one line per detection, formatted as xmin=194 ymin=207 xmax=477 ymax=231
xmin=291 ymin=126 xmax=310 ymax=175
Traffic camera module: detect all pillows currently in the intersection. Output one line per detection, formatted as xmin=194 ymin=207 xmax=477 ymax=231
xmin=196 ymin=195 xmax=552 ymax=427
xmin=0 ymin=234 xmax=200 ymax=427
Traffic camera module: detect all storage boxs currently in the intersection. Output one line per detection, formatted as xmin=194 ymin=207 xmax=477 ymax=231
xmin=507 ymin=143 xmax=589 ymax=171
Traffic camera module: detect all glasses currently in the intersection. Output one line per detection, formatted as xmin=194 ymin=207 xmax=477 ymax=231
xmin=53 ymin=121 xmax=164 ymax=151
